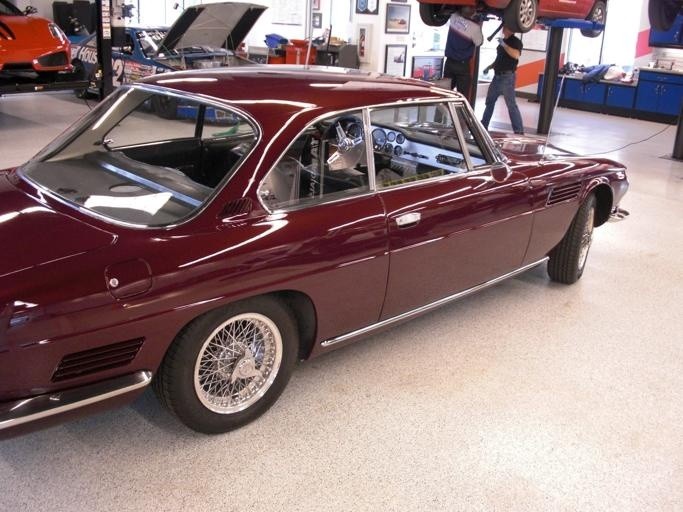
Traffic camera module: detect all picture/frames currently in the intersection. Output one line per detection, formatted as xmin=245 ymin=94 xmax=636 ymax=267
xmin=384 ymin=2 xmax=412 ymax=35
xmin=410 ymin=56 xmax=444 ymax=81
xmin=312 ymin=12 xmax=322 ymax=29
xmin=312 ymin=0 xmax=320 ymax=10
xmin=384 ymin=44 xmax=408 ymax=77
xmin=354 ymin=0 xmax=379 ymax=16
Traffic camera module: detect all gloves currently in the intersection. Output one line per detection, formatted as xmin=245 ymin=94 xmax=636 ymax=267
xmin=496 ymin=37 xmax=505 ymax=46
xmin=483 ymin=67 xmax=490 ymax=75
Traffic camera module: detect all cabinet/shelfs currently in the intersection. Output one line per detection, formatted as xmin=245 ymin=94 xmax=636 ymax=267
xmin=536 ymin=72 xmax=564 ymax=99
xmin=607 ymin=82 xmax=637 ymax=111
xmin=564 ymin=78 xmax=608 ymax=106
xmin=633 ymin=66 xmax=683 ymax=118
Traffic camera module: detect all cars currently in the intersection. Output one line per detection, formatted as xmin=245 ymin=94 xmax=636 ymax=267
xmin=417 ymin=1 xmax=608 ymax=37
xmin=0 ymin=62 xmax=630 ymax=438
xmin=0 ymin=1 xmax=72 ymax=81
xmin=647 ymin=0 xmax=682 ymax=32
xmin=70 ymin=3 xmax=270 ymax=126
xmin=395 ymin=18 xmax=406 ymax=25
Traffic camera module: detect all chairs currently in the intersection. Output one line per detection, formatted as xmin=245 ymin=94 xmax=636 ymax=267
xmin=287 ymin=156 xmax=361 ymax=198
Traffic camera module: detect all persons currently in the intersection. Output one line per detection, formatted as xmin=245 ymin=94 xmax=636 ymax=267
xmin=480 ymin=25 xmax=524 ymax=135
xmin=443 ymin=5 xmax=484 ymax=104
